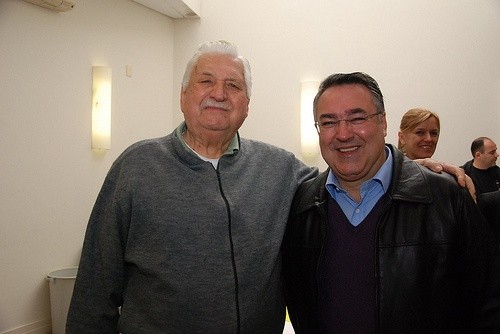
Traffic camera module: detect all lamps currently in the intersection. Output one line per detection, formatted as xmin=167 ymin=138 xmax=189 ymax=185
xmin=89 ymin=66 xmax=113 ymax=157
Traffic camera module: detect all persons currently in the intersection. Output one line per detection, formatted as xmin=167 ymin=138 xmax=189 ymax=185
xmin=65 ymin=39 xmax=478 ymax=334
xmin=281 ymin=72 xmax=500 ymax=334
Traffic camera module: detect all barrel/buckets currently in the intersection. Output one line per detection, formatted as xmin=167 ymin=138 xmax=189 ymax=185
xmin=44 ymin=267 xmax=78 ymax=334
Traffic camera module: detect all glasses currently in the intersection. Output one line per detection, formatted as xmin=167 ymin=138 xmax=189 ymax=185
xmin=315 ymin=111 xmax=382 ymax=134
